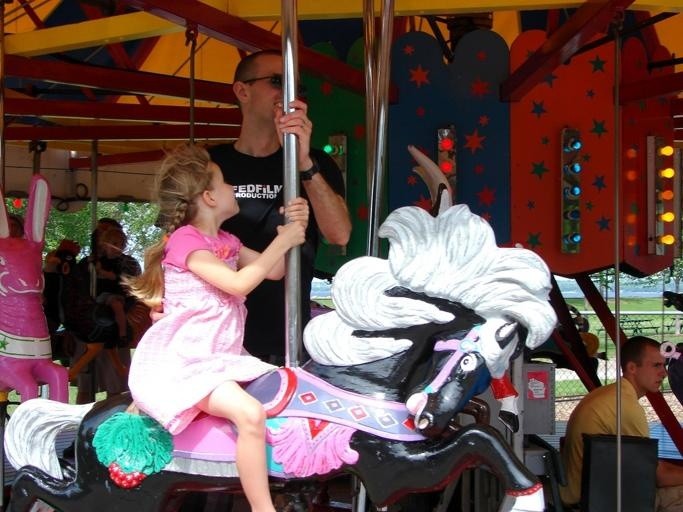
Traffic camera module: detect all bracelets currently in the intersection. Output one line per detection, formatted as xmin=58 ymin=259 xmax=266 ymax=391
xmin=298 ymin=163 xmax=318 ymax=181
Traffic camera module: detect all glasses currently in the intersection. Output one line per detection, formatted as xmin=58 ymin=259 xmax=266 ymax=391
xmin=242 ymin=76 xmax=282 ymax=88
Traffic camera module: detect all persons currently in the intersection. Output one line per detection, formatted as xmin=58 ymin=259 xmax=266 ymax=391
xmin=8 ymin=212 xmax=24 ymax=238
xmin=126 ymin=142 xmax=309 ymax=511
xmin=61 ymin=217 xmax=142 ymax=398
xmin=561 ymin=336 xmax=683 ymax=511
xmin=153 ymin=51 xmax=353 ymax=369
